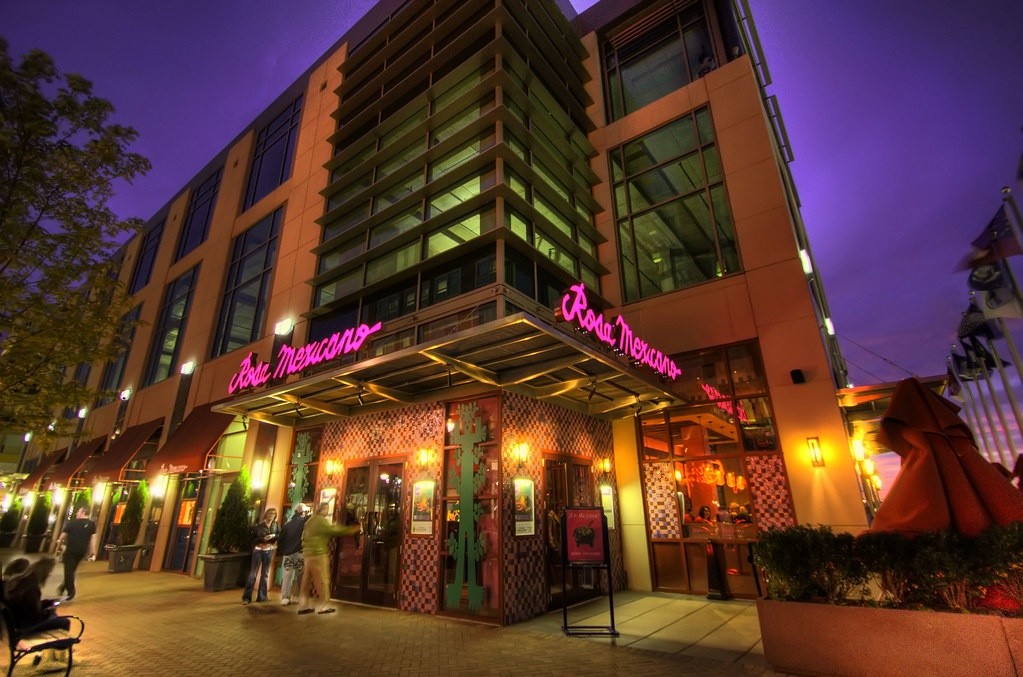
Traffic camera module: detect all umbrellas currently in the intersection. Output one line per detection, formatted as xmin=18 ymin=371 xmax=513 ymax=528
xmin=854 ymin=374 xmax=1022 ymax=537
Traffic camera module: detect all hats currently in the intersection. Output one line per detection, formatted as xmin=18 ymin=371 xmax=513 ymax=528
xmin=295 ymin=503 xmax=310 ymax=513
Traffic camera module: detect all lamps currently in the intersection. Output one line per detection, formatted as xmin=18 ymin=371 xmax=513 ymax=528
xmin=517 ymin=442 xmax=528 ymax=468
xmin=325 ymin=458 xmax=333 ymax=480
xmin=602 ymin=456 xmax=611 ymax=476
xmin=78 ymin=408 xmax=87 ymax=418
xmin=419 ymin=447 xmax=428 ymax=471
xmin=275 ymin=319 xmax=293 ymax=336
xmin=803 ymin=437 xmax=825 ymax=468
xmin=120 ymin=390 xmax=130 ymax=401
xmin=24 ymin=433 xmax=32 ymax=441
xmin=181 ymin=363 xmax=194 ymax=375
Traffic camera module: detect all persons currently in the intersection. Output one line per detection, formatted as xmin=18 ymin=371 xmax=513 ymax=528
xmin=682 ymin=497 xmax=751 ymax=525
xmin=52 ymin=506 xmax=98 ymax=602
xmin=241 ymin=502 xmax=403 ymax=614
xmin=1 ymin=557 xmax=75 ymax=662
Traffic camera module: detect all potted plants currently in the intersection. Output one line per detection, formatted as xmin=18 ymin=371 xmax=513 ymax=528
xmin=197 ymin=463 xmax=253 ymax=592
xmin=22 ymin=493 xmax=53 ymax=554
xmin=749 ymin=522 xmax=1023 ymax=677
xmin=70 ymin=489 xmax=92 ymax=562
xmin=102 ymin=479 xmax=149 ymax=572
xmin=0 ymin=499 xmax=19 ymax=546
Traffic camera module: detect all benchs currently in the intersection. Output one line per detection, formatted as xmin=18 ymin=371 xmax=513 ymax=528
xmin=1 ymin=604 xmax=85 ymax=677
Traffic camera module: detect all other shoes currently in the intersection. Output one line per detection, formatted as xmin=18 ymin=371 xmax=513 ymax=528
xmin=35 ymin=649 xmax=69 ymax=672
xmin=297 ymin=608 xmax=315 ymax=614
xmin=242 ymin=598 xmax=250 ymax=605
xmin=57 ymin=585 xmax=65 ymax=595
xmin=281 ymin=597 xmax=289 ymax=605
xmin=66 ymin=592 xmax=76 ymax=601
xmin=318 ymin=608 xmax=336 ymax=614
xmin=57 ymin=648 xmax=81 ymax=666
